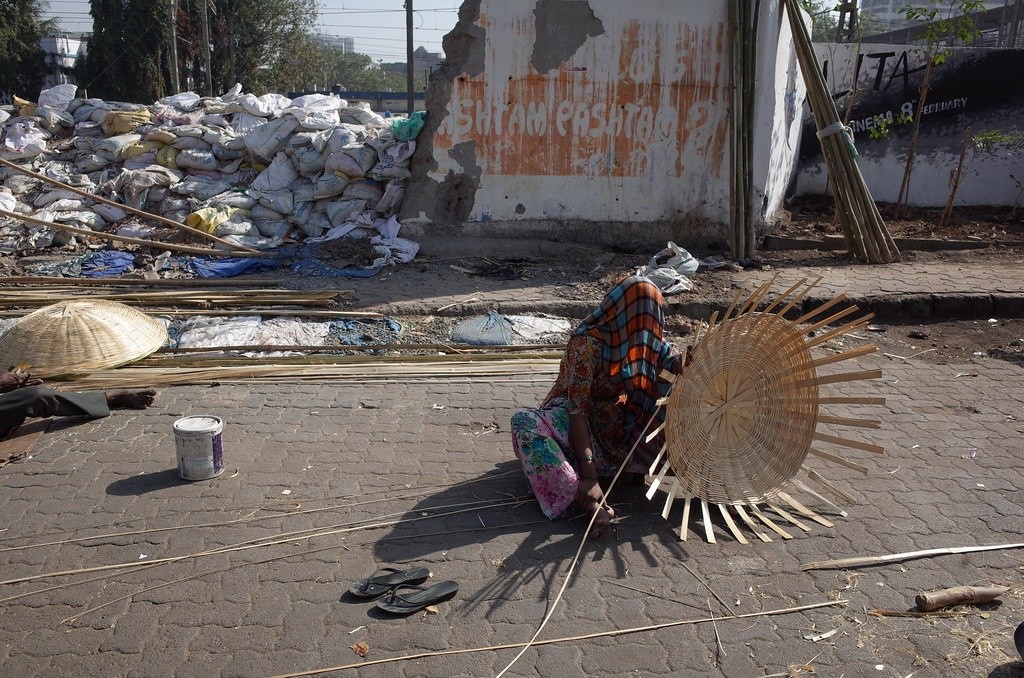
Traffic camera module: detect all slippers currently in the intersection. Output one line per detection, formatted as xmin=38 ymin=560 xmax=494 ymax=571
xmin=378 ymin=580 xmax=459 ymax=616
xmin=349 ymin=567 xmax=429 ymax=599
xmin=587 ymin=516 xmax=620 ymax=540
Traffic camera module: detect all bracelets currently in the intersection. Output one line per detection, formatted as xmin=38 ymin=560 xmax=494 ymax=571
xmin=579 ymin=454 xmax=598 ymax=480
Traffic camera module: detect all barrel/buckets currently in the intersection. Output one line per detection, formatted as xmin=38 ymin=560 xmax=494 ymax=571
xmin=173 ymin=414 xmax=240 ymax=480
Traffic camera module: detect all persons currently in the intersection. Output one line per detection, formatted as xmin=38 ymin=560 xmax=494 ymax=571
xmin=0 ymin=367 xmax=156 ymax=435
xmin=510 ymin=276 xmax=693 ymax=541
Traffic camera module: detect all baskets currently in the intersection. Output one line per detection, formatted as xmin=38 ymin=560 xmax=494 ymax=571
xmin=645 ymin=272 xmax=886 ymax=544
xmin=0 ymin=298 xmax=167 ymax=377
